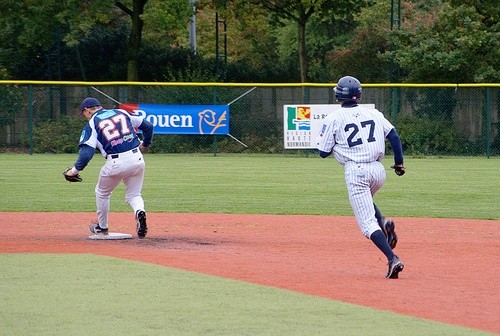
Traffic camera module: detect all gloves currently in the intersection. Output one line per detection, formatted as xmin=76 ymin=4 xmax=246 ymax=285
xmin=394 ymin=164 xmax=405 ymax=176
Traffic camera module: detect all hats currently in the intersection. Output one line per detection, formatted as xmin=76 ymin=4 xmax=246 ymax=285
xmin=78 ymin=97 xmax=102 ymax=117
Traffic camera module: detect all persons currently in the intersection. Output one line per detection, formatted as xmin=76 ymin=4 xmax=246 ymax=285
xmin=66 ymin=97 xmax=152 ymax=239
xmin=318 ymin=75 xmax=406 ymax=279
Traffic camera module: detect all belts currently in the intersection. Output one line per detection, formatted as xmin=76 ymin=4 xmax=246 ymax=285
xmin=106 ymin=148 xmax=138 ymax=159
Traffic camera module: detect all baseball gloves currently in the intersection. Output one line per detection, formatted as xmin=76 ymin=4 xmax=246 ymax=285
xmin=62 ymin=168 xmax=83 ymax=183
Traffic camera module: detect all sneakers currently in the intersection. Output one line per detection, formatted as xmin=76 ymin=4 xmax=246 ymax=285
xmin=89 ymin=224 xmax=108 ymax=236
xmin=380 ymin=218 xmax=397 ymax=249
xmin=134 ymin=209 xmax=147 ymax=238
xmin=386 ymin=254 xmax=404 ymax=279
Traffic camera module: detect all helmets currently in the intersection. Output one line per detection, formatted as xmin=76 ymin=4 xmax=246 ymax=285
xmin=333 ymin=75 xmax=362 ymax=102
